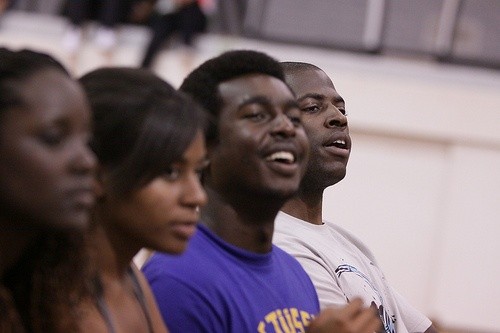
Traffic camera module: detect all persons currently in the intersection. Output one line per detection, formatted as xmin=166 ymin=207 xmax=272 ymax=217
xmin=271 ymin=61 xmax=437 ymax=333
xmin=0 ymin=46 xmax=100 ymax=333
xmin=140 ymin=49 xmax=382 ymax=333
xmin=72 ymin=65 xmax=211 ymax=333
